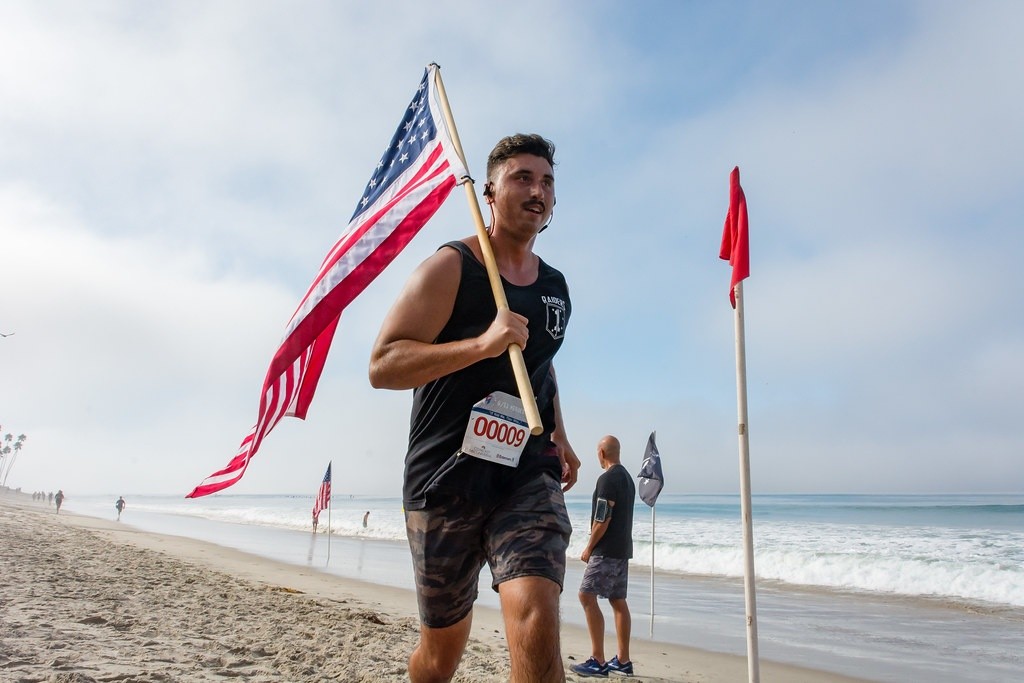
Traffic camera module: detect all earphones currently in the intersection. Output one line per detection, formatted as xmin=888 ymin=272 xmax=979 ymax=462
xmin=483 ymin=185 xmax=492 ymax=197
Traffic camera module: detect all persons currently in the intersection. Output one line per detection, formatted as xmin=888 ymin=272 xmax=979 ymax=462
xmin=116 ymin=496 xmax=125 ymax=521
xmin=368 ymin=134 xmax=581 ymax=683
xmin=32 ymin=490 xmax=64 ymax=514
xmin=363 ymin=511 xmax=370 ymax=528
xmin=568 ymin=435 xmax=635 ymax=679
xmin=312 ymin=516 xmax=319 ymax=535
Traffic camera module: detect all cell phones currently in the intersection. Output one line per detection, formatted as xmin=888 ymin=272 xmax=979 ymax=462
xmin=594 ymin=498 xmax=607 ymax=522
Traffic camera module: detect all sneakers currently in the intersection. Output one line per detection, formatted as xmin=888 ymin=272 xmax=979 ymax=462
xmin=606 ymin=655 xmax=634 ymax=676
xmin=569 ymin=656 xmax=609 ymax=678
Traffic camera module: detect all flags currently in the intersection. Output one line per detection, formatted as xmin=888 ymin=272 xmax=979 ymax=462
xmin=715 ymin=168 xmax=750 ymax=310
xmin=185 ymin=66 xmax=465 ymax=497
xmin=637 ymin=431 xmax=664 ymax=507
xmin=312 ymin=463 xmax=330 ymax=520
